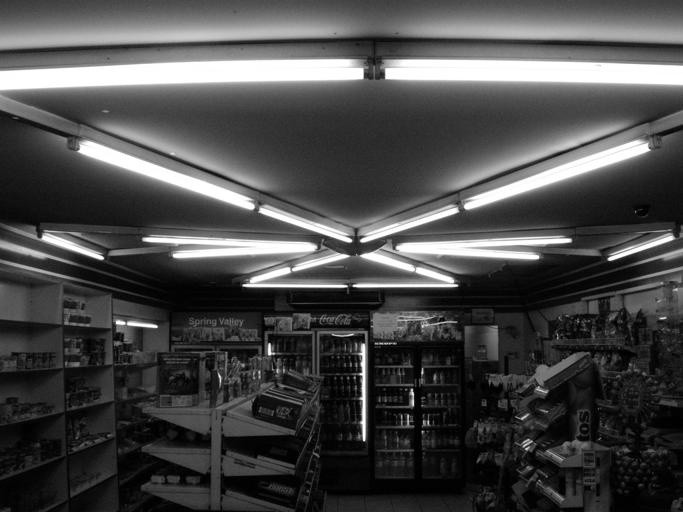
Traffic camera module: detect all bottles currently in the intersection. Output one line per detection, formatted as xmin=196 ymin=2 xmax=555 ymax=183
xmin=320 ymin=334 xmax=363 ymax=447
xmin=420 ymin=345 xmax=460 ymax=478
xmin=267 ymin=336 xmax=312 ymax=380
xmin=374 ymin=347 xmax=416 ymax=479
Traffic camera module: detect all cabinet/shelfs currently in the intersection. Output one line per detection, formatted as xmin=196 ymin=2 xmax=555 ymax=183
xmin=467 ymin=313 xmax=683 ymax=512
xmin=174 ymin=326 xmax=330 ymax=511
xmin=2 ymin=260 xmax=173 ymax=511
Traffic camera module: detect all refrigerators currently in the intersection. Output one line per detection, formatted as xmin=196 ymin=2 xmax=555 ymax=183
xmin=263 ymin=329 xmax=369 ymax=494
xmin=370 ymin=338 xmax=468 ymax=495
